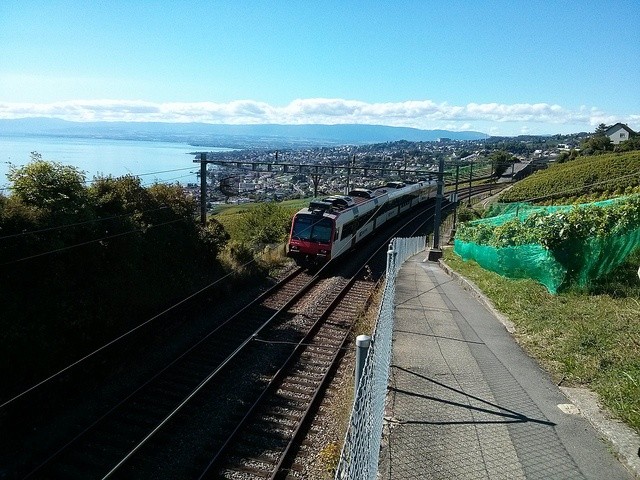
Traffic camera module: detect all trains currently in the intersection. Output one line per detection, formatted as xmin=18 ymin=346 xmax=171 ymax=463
xmin=288 ymin=175 xmax=438 ymax=267
